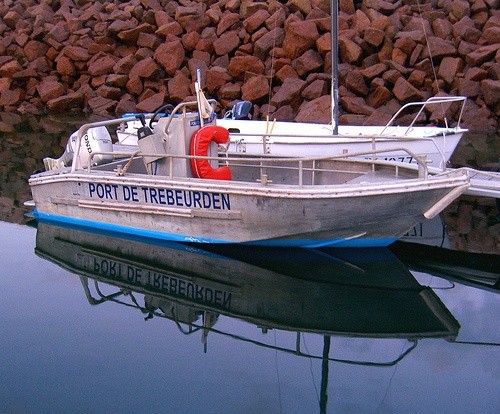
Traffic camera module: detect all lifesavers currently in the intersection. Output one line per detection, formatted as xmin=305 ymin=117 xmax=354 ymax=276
xmin=189 ymin=125 xmax=231 ymax=180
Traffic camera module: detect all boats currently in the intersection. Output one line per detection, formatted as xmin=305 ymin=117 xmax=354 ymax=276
xmin=23 ymin=68 xmax=470 ymax=247
xmin=115 ymin=0 xmax=469 ymax=171
xmin=34 ymin=223 xmax=500 ymax=414
